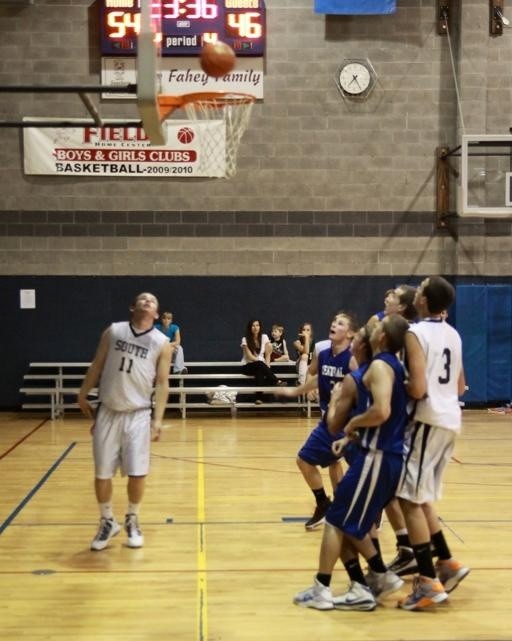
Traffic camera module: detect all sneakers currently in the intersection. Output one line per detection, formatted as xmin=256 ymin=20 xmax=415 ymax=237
xmin=385 ymin=546 xmax=420 ymax=577
xmin=180 ymin=367 xmax=188 ymax=374
xmin=364 ymin=566 xmax=404 ymax=602
xmin=255 ymin=399 xmax=263 ymax=404
xmin=305 ymin=496 xmax=333 ymax=529
xmin=293 ymin=575 xmax=334 ymax=610
xmin=434 ymin=556 xmax=470 ymax=593
xmin=398 ymin=573 xmax=448 ymax=611
xmin=276 ymin=380 xmax=288 ymax=386
xmin=333 ymin=581 xmax=378 ymax=611
xmin=90 ymin=516 xmax=121 ymax=551
xmin=123 ymin=513 xmax=144 ymax=548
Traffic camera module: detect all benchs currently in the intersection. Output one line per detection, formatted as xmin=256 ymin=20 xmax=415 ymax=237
xmin=15 ymin=361 xmax=323 ymax=418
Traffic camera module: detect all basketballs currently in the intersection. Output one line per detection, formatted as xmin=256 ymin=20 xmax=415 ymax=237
xmin=199 ymin=39 xmax=235 ymax=78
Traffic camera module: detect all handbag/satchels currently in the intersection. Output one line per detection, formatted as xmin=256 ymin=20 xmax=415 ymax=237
xmin=206 ymin=384 xmax=238 ymax=405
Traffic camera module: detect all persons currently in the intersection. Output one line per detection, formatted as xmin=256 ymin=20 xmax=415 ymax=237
xmin=268 ymin=323 xmax=291 ymax=362
xmin=293 ymin=274 xmax=473 ymax=615
xmin=151 ymin=311 xmax=188 ymax=375
xmin=239 ymin=317 xmax=288 ymax=404
xmin=78 ymin=291 xmax=173 ymax=551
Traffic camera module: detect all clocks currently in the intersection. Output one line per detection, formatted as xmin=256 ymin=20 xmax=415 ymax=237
xmin=338 ymin=61 xmax=371 ymax=96
